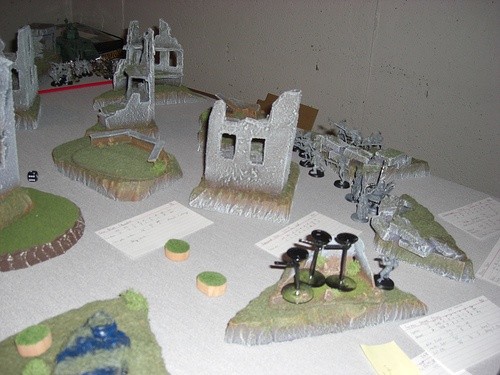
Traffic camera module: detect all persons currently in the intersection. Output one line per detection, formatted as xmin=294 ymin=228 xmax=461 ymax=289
xmin=295 ymin=124 xmax=394 ymax=220
xmin=377 ymin=254 xmax=397 ymax=283
xmin=48 ymin=54 xmax=115 ymax=85
